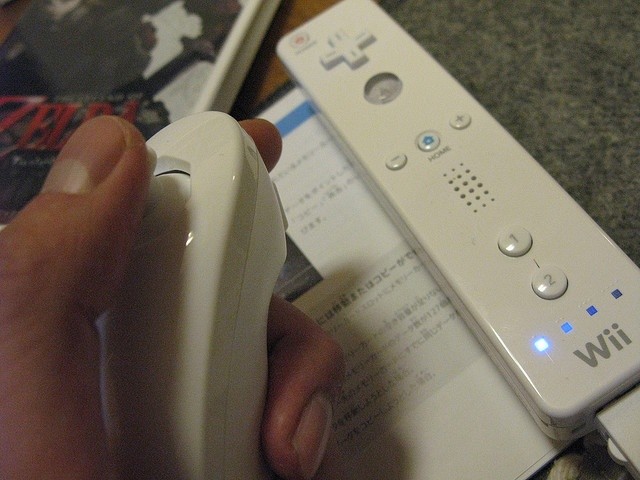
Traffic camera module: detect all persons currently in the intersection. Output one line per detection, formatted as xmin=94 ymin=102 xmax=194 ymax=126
xmin=0 ymin=115 xmax=343 ymax=480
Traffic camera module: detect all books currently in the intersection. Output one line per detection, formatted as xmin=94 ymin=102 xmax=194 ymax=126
xmin=1 ymin=0 xmax=285 ymax=228
